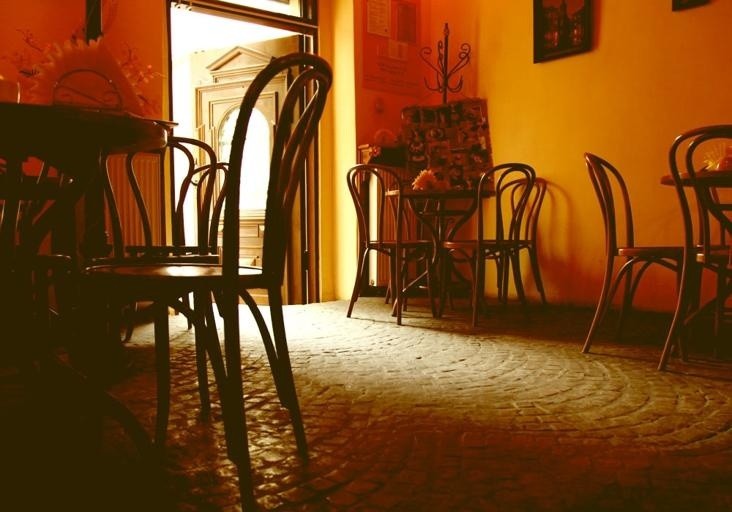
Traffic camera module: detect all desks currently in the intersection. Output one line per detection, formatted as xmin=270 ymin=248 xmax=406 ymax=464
xmin=2 ymin=99 xmax=170 ymax=463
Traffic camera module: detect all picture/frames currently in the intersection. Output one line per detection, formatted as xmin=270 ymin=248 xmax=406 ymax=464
xmin=530 ymin=0 xmax=593 ymax=63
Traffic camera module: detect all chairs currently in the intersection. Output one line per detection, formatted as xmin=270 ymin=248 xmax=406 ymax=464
xmin=98 ymin=135 xmax=229 ymax=448
xmin=344 ymin=161 xmax=547 ymax=328
xmin=81 ymin=52 xmax=334 ymax=480
xmin=581 ymin=123 xmax=732 ymax=370
xmin=126 ymin=161 xmax=229 ymax=341
